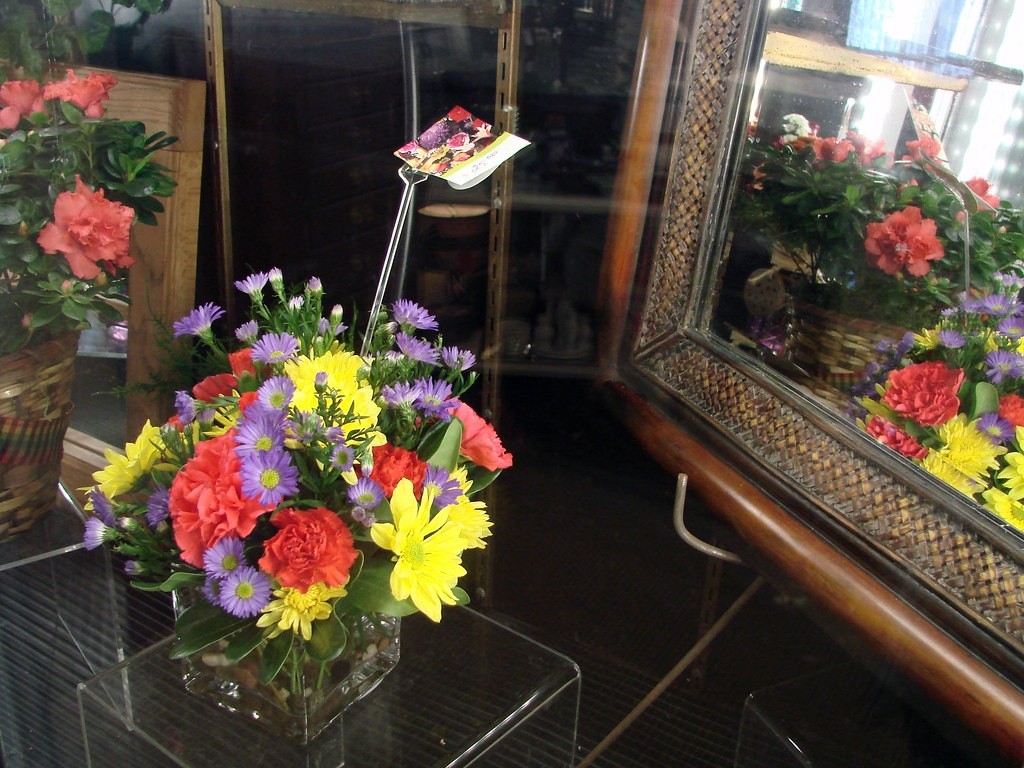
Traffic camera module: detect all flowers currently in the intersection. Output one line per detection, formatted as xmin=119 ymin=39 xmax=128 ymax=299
xmin=81 ymin=266 xmax=519 ymax=698
xmin=729 ymin=113 xmax=1024 ymax=534
xmin=0 ymin=0 xmax=180 ymax=352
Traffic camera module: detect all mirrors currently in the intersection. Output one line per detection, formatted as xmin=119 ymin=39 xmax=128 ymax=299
xmin=0 ymin=56 xmax=208 ymax=459
xmin=591 ymin=1 xmax=1024 ymax=768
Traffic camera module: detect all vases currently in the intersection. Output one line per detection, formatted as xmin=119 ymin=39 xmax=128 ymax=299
xmin=168 ymin=557 xmax=403 ymax=748
